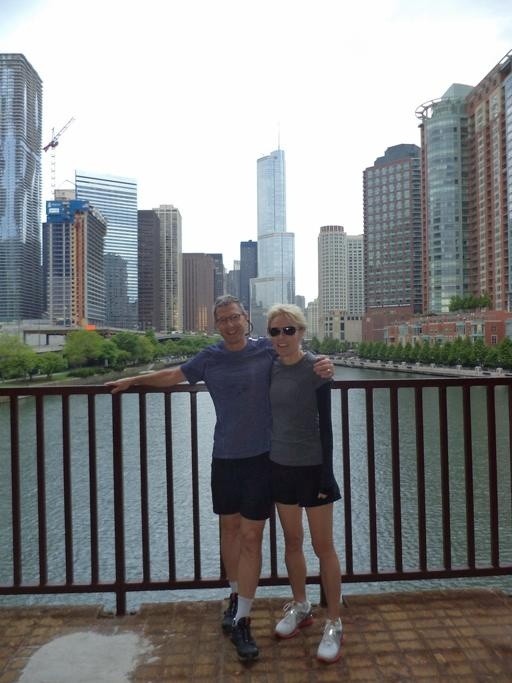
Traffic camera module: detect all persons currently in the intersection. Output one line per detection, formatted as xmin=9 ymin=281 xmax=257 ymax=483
xmin=104 ymin=296 xmax=332 ymax=661
xmin=266 ymin=303 xmax=345 ymax=665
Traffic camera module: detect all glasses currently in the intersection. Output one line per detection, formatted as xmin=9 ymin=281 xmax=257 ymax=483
xmin=215 ymin=313 xmax=241 ymax=324
xmin=269 ymin=326 xmax=297 ymax=337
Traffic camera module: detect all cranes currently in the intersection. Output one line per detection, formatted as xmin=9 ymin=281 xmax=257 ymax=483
xmin=42 ymin=117 xmax=77 ymax=200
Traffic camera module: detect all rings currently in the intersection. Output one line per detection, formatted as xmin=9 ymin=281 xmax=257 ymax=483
xmin=328 ymin=369 xmax=330 ymax=372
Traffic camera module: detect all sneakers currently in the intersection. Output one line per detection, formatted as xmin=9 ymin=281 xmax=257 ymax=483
xmin=316 ymin=617 xmax=345 ymax=665
xmin=273 ymin=600 xmax=314 ymax=638
xmin=222 ymin=592 xmax=259 ymax=658
